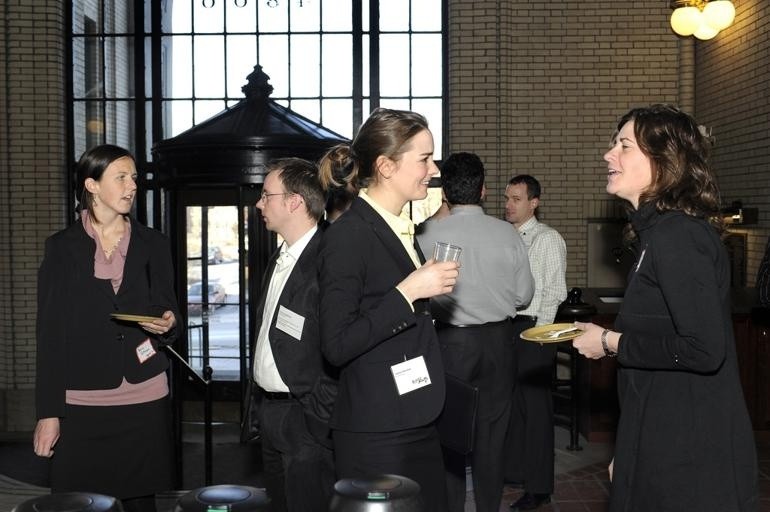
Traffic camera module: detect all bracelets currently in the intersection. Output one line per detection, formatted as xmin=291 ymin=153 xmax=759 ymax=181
xmin=601 ymin=327 xmax=617 ymax=358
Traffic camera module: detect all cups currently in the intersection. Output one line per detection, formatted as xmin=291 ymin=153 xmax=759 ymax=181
xmin=433 ymin=242 xmax=463 ymax=266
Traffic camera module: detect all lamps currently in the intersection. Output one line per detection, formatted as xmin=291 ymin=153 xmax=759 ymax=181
xmin=670 ymin=0 xmax=736 ymax=41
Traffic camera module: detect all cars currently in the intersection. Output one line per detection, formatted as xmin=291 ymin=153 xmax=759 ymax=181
xmin=187 ymin=282 xmax=227 ymax=314
xmin=192 ymin=245 xmax=223 ymax=266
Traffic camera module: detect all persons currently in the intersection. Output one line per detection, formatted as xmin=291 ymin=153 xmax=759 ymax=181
xmin=236 ymin=108 xmax=569 ymax=511
xmin=571 ymin=102 xmax=763 ymax=510
xmin=31 ymin=142 xmax=188 ymax=512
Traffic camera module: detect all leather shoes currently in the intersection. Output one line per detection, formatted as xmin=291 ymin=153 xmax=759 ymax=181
xmin=505 ymin=476 xmax=522 ymax=486
xmin=510 ymin=493 xmax=552 ymax=510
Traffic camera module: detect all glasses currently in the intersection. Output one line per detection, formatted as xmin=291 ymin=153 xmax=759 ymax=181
xmin=260 ymin=192 xmax=291 ymax=205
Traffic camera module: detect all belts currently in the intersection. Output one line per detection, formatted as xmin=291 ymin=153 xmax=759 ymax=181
xmin=250 ymin=382 xmax=293 ymax=400
xmin=452 ymin=320 xmax=512 ymax=328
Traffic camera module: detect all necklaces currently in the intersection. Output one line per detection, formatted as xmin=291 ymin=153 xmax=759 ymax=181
xmin=99 ymin=222 xmax=127 ymax=255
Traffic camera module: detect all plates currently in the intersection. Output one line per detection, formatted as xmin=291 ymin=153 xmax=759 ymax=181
xmin=110 ymin=313 xmax=161 ymax=322
xmin=520 ymin=323 xmax=585 ymax=344
